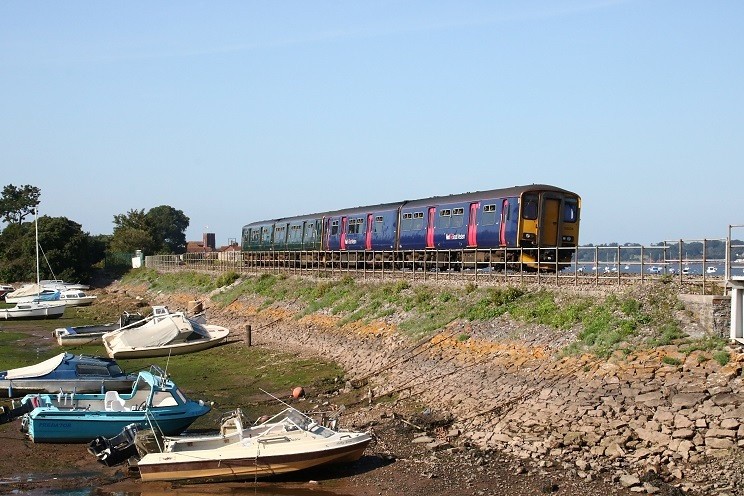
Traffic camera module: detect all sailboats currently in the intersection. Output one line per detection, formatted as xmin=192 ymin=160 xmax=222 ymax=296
xmin=0 ymin=207 xmax=97 ymax=306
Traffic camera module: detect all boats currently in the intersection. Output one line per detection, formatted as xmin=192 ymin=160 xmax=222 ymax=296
xmin=101 ymin=311 xmax=230 ymax=359
xmin=706 ymin=266 xmax=716 ymax=274
xmin=55 ymin=309 xmax=149 ymax=347
xmin=0 ymin=350 xmax=141 ymax=394
xmin=0 ymin=301 xmax=67 ymax=319
xmin=576 ymin=252 xmax=692 ymax=275
xmin=17 ymin=364 xmax=211 ymax=443
xmin=138 ymin=388 xmax=372 ymax=484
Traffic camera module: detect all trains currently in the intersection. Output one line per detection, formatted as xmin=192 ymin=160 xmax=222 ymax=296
xmin=240 ymin=183 xmax=582 ymax=274
